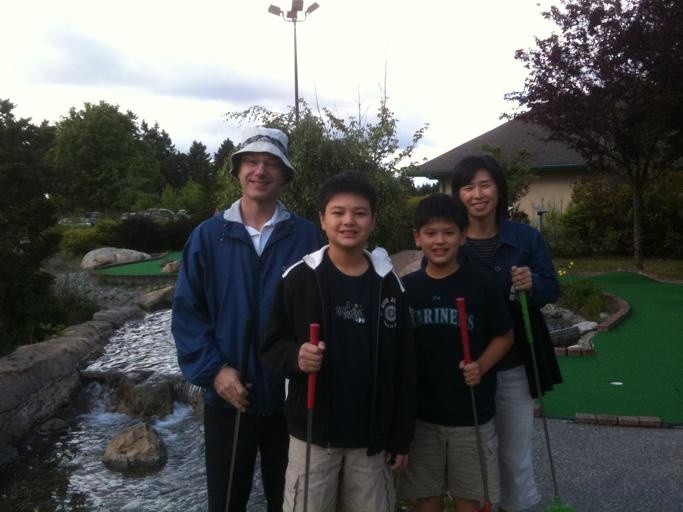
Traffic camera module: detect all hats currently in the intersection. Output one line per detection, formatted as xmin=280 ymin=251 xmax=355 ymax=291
xmin=230 ymin=127 xmax=296 ymax=184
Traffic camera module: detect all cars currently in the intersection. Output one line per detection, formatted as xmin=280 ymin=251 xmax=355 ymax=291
xmin=57 ymin=212 xmax=104 ymax=227
xmin=121 ymin=207 xmax=192 ymax=227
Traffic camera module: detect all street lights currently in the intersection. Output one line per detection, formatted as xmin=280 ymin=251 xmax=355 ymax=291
xmin=268 ymin=0 xmax=319 ymax=127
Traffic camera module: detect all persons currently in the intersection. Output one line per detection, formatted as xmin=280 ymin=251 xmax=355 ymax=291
xmin=396 ymin=189 xmax=517 ymax=511
xmin=266 ymin=166 xmax=417 ymax=509
xmin=172 ymin=127 xmax=327 ymax=509
xmin=418 ymin=151 xmax=563 ymax=509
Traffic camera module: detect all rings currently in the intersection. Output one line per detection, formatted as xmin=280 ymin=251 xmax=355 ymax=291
xmin=521 ymin=280 xmax=526 ymax=284
